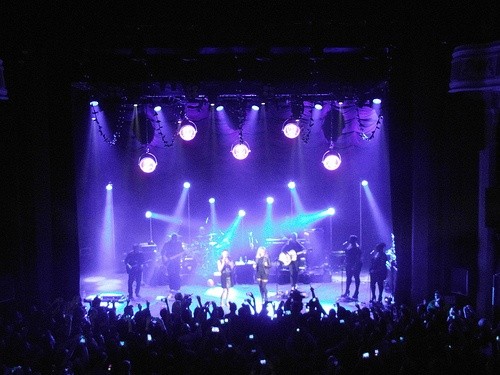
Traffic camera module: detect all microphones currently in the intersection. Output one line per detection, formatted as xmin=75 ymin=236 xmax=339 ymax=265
xmin=343 ymin=241 xmax=348 ymax=245
xmin=370 ymin=250 xmax=374 ymax=255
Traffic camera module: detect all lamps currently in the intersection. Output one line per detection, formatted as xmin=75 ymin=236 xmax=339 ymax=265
xmin=137 ymin=96 xmax=158 ymax=174
xmin=322 ymin=100 xmax=342 ymax=171
xmin=230 ymin=92 xmax=251 ymax=160
xmin=175 ymin=100 xmax=197 ymax=141
xmin=282 ymin=96 xmax=301 ymax=139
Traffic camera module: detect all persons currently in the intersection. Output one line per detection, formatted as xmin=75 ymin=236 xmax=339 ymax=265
xmin=0 ymin=232 xmax=500 ymax=375
